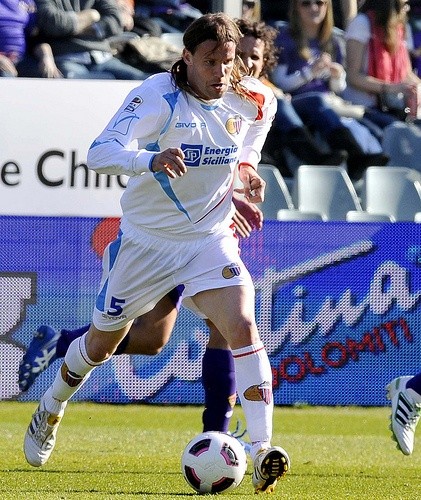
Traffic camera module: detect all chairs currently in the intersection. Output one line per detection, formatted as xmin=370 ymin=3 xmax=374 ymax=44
xmin=160 ymin=32 xmax=421 ymax=220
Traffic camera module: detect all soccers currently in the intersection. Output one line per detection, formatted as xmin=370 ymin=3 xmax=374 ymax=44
xmin=180 ymin=430 xmax=248 ymax=495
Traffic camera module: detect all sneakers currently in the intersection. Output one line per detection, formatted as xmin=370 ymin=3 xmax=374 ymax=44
xmin=384 ymin=375 xmax=421 ymax=456
xmin=23 ymin=390 xmax=64 ymax=467
xmin=18 ymin=325 xmax=62 ymax=392
xmin=252 ymin=445 xmax=290 ymax=494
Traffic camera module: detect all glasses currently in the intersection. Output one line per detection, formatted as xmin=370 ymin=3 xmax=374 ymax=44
xmin=302 ymin=0 xmax=327 ymax=7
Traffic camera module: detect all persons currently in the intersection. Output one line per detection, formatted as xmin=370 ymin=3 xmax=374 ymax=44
xmin=345 ymin=0 xmax=421 ymax=140
xmin=0 ymin=0 xmax=203 ymax=80
xmin=15 ymin=17 xmax=279 ymax=451
xmin=268 ymin=0 xmax=390 ymax=182
xmin=23 ymin=11 xmax=290 ymax=494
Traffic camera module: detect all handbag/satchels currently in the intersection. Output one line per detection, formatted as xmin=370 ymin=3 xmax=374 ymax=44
xmin=378 ymin=93 xmax=408 ymax=120
